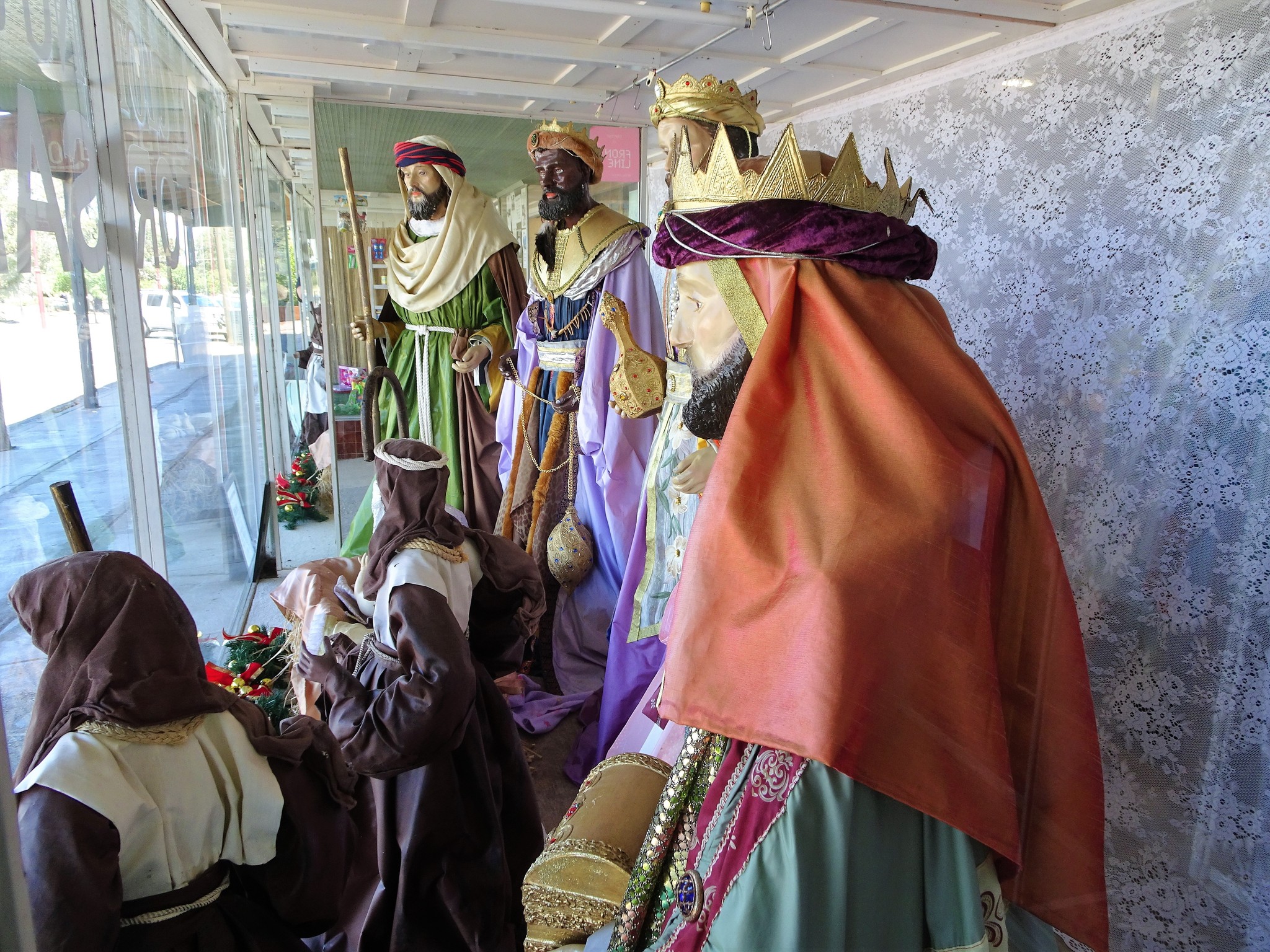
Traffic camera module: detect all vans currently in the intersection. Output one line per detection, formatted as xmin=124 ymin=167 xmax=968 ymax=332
xmin=138 ymin=288 xmax=227 ymax=341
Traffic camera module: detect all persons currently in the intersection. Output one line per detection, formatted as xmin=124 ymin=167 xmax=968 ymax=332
xmin=493 ymin=116 xmax=672 ymax=736
xmin=299 ymin=437 xmax=542 ymax=952
xmin=292 ymin=303 xmax=328 ymax=451
xmin=295 ymin=276 xmax=303 ymax=303
xmin=7 ymin=551 xmax=296 ymax=950
xmin=519 ymin=123 xmax=1112 ymax=952
xmin=342 ymin=134 xmax=530 ymax=556
xmin=566 ymin=67 xmax=764 ymax=786
